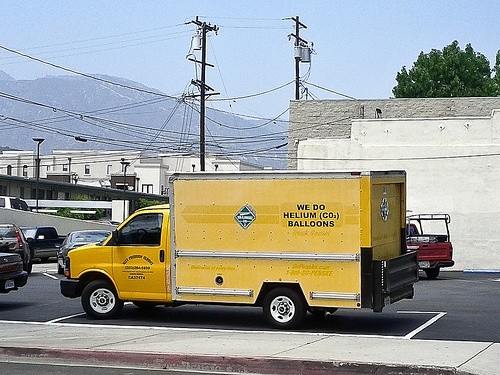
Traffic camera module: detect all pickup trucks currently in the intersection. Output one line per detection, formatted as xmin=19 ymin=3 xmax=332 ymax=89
xmin=18 ymin=225 xmax=67 ymax=264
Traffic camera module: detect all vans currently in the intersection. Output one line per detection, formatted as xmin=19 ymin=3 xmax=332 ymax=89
xmin=0 ymin=195 xmax=33 ymax=214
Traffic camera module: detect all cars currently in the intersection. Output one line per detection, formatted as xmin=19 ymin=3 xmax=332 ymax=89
xmin=0 ymin=253 xmax=29 ymax=295
xmin=57 ymin=230 xmax=112 ymax=273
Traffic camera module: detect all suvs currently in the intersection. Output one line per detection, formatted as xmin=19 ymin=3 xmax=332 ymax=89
xmin=0 ymin=223 xmax=34 ymax=277
xmin=405 ymin=209 xmax=455 ymax=280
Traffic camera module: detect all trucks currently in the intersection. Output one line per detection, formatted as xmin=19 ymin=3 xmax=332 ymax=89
xmin=60 ymin=170 xmax=413 ymax=329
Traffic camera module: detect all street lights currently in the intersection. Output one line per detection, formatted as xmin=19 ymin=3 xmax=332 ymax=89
xmin=120 ymin=161 xmax=131 ymax=222
xmin=31 ymin=137 xmax=45 ymax=213
